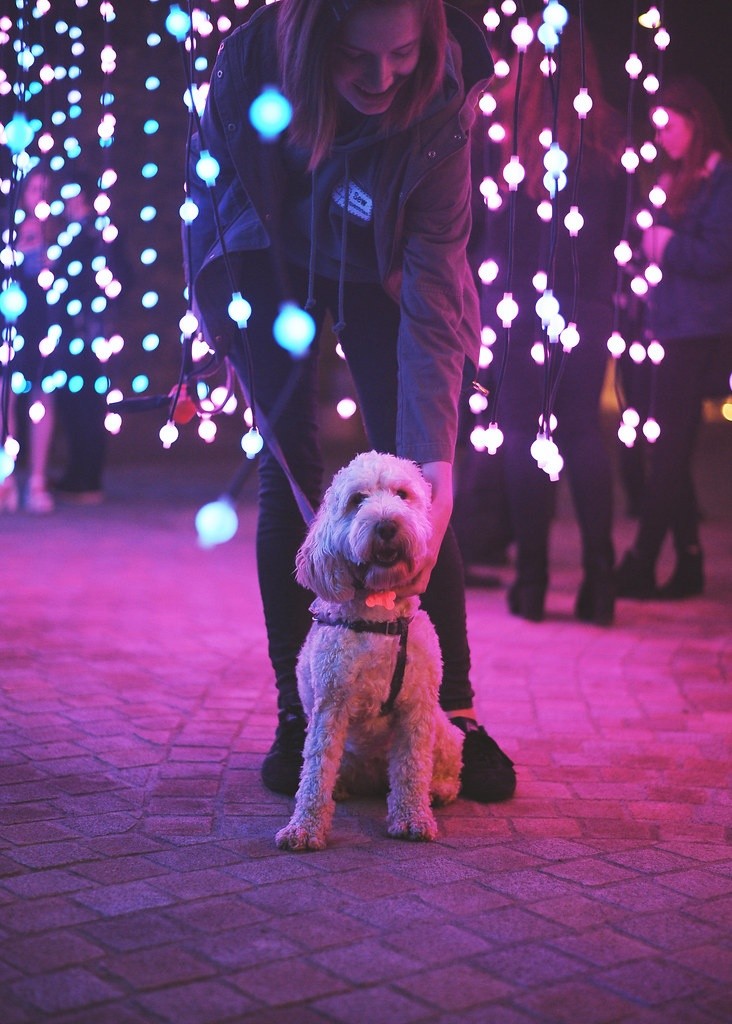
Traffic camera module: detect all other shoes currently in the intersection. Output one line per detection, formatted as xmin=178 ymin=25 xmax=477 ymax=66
xmin=28 ymin=492 xmax=53 ymax=513
xmin=56 ymin=488 xmax=103 ymax=505
xmin=449 ymin=716 xmax=516 ymax=802
xmin=507 ymin=565 xmax=547 ymax=619
xmin=261 ymin=710 xmax=308 ymax=795
xmin=660 ymin=552 xmax=705 ymax=597
xmin=615 ymin=550 xmax=657 ymax=598
xmin=3 ymin=475 xmax=19 ymax=513
xmin=576 ymin=573 xmax=615 ymax=625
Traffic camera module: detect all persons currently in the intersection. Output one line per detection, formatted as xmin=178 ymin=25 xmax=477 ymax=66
xmin=449 ymin=12 xmax=732 ymax=625
xmin=0 ymin=175 xmax=112 ymax=515
xmin=184 ymin=0 xmax=518 ymax=804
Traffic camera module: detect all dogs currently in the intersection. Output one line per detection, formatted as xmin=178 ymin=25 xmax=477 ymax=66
xmin=273 ymin=446 xmax=470 ymax=855
